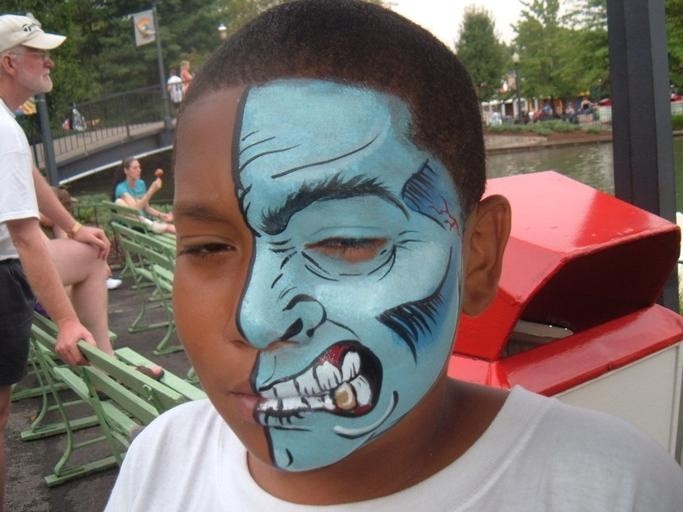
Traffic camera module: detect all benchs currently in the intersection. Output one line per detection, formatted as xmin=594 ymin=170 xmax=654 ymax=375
xmin=10 ymin=200 xmax=209 ymax=487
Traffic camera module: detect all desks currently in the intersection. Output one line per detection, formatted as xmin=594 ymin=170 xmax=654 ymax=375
xmin=509 ymin=53 xmax=525 ymax=123
xmin=215 ymin=23 xmax=228 ymax=43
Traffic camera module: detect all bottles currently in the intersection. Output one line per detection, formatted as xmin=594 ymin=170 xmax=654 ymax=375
xmin=0 ymin=13 xmax=67 ymax=50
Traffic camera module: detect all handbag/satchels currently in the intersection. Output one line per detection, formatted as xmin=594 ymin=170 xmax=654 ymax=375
xmin=68 ymin=221 xmax=81 ymax=239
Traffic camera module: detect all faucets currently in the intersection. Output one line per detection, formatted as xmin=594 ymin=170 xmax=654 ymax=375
xmin=89 ymin=365 xmax=165 ymax=402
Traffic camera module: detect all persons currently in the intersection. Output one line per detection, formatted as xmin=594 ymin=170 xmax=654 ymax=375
xmin=167 ymin=67 xmax=183 ymax=112
xmin=104 ymin=1 xmax=682 ymax=512
xmin=0 ymin=14 xmax=166 ymax=512
xmin=580 ymin=96 xmax=592 ymax=112
xmin=179 ymin=59 xmax=194 ymax=94
xmin=111 ymin=157 xmax=175 ymax=234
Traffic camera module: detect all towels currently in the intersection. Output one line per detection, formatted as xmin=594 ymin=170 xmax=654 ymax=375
xmin=444 ymin=169 xmax=683 ymax=463
